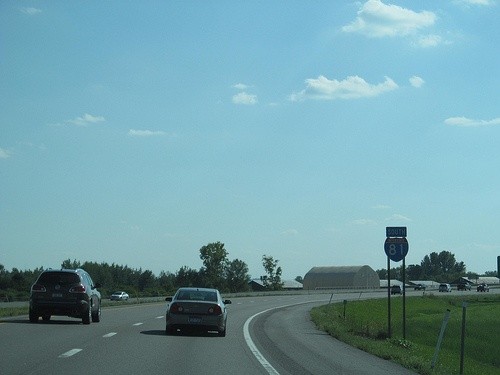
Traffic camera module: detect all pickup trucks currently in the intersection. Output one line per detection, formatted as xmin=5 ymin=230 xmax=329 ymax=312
xmin=477 ymin=283 xmax=489 ymax=292
xmin=458 ymin=282 xmax=471 ymax=291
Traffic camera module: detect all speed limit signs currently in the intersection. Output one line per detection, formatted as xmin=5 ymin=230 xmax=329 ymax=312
xmin=383 ymin=227 xmax=409 ymax=263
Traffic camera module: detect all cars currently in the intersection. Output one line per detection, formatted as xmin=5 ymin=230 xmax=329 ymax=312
xmin=165 ymin=286 xmax=231 ymax=337
xmin=110 ymin=291 xmax=128 ymax=300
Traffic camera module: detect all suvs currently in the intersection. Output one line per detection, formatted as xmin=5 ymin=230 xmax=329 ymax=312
xmin=391 ymin=285 xmax=403 ymax=295
xmin=29 ymin=267 xmax=101 ymax=324
xmin=414 ymin=283 xmax=426 ymax=290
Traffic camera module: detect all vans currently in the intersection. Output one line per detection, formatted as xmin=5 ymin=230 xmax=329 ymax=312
xmin=439 ymin=284 xmax=452 ymax=292
